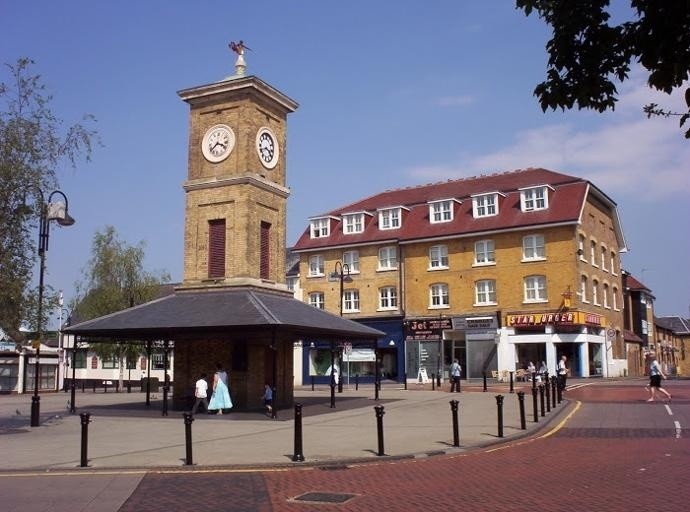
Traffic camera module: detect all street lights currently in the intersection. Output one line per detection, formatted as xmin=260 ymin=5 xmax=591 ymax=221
xmin=14 ymin=185 xmax=75 ymax=428
xmin=328 ymin=261 xmax=353 ymax=393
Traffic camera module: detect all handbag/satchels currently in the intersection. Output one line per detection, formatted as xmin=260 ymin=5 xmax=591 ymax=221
xmin=449 ymin=378 xmax=455 ymax=384
xmin=645 ymin=384 xmax=651 ymax=392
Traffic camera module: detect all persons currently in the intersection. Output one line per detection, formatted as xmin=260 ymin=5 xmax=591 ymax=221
xmin=330 ymin=368 xmax=339 ymax=388
xmin=645 ymin=353 xmax=672 ymax=402
xmin=260 ymin=383 xmax=273 ymax=406
xmin=556 ymin=356 xmax=569 ymax=392
xmin=208 ymin=362 xmax=233 ymax=415
xmin=449 ymin=359 xmax=462 ymax=393
xmin=532 ymin=361 xmax=547 ymax=381
xmin=191 ymin=373 xmax=211 ymax=415
xmin=528 ymin=361 xmax=535 ymax=381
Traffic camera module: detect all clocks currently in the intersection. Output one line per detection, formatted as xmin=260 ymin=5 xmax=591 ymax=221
xmin=201 ymin=124 xmax=235 ymax=163
xmin=255 ymin=126 xmax=279 ymax=169
